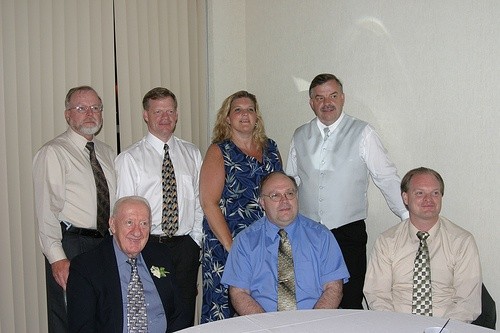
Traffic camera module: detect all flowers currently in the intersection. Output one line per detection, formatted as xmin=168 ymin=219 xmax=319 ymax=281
xmin=149 ymin=266 xmax=168 ymax=278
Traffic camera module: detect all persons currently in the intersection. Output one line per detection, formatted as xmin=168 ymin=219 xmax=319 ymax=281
xmin=198 ymin=91 xmax=283 ymax=323
xmin=221 ymin=172 xmax=350 ymax=315
xmin=287 ymin=74 xmax=409 ymax=310
xmin=66 ymin=196 xmax=186 ymax=333
xmin=363 ymin=167 xmax=482 ymax=325
xmin=32 ymin=86 xmax=118 ymax=333
xmin=113 ymin=87 xmax=205 ymax=328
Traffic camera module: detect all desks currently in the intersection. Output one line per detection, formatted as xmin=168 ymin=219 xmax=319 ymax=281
xmin=172 ymin=309 xmax=500 ymax=333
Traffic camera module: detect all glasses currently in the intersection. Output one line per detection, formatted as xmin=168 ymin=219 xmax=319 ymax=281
xmin=263 ymin=191 xmax=296 ymax=202
xmin=67 ymin=104 xmax=102 ymax=114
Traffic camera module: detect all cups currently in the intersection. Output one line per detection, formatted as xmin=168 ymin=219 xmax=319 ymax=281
xmin=424 ymin=327 xmax=451 ymax=333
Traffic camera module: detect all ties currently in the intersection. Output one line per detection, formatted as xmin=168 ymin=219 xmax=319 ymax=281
xmin=126 ymin=258 xmax=148 ymax=333
xmin=162 ymin=144 xmax=179 ymax=239
xmin=277 ymin=229 xmax=298 ymax=311
xmin=85 ymin=141 xmax=110 ymax=237
xmin=411 ymin=231 xmax=433 ymax=317
xmin=324 ymin=128 xmax=330 ymax=141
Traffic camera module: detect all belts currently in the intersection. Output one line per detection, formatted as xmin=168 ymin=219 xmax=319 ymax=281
xmin=62 ymin=225 xmax=105 ymax=238
xmin=150 ymin=235 xmax=190 ymax=243
